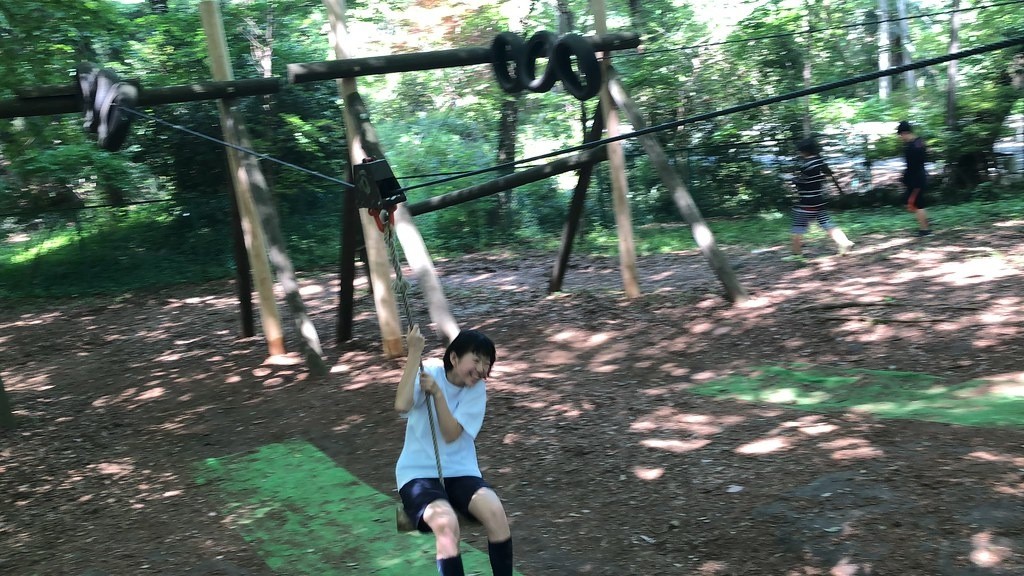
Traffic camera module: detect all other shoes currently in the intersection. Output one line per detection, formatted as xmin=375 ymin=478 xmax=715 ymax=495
xmin=781 ymin=254 xmax=804 ymax=261
xmin=837 ymin=242 xmax=855 ymax=257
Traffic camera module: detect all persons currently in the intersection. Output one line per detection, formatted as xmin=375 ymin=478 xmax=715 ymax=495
xmin=780 ymin=139 xmax=854 ymax=261
xmin=896 ymin=121 xmax=934 ymax=236
xmin=395 ymin=324 xmax=514 ymax=576
